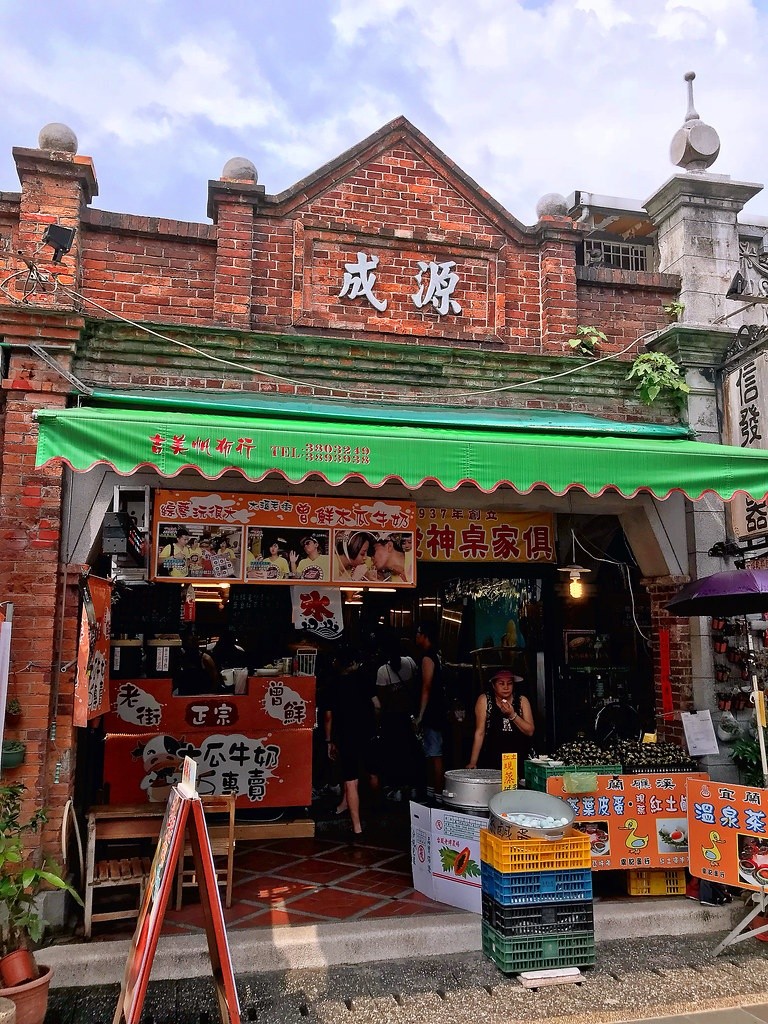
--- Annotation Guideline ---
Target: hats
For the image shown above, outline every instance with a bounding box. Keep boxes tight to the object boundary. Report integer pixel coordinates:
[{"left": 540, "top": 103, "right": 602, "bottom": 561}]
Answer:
[
  {"left": 300, "top": 536, "right": 317, "bottom": 546},
  {"left": 488, "top": 669, "right": 524, "bottom": 682}
]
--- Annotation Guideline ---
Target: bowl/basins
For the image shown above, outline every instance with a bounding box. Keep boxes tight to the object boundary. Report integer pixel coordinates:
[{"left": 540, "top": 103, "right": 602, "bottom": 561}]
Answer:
[
  {"left": 739, "top": 845, "right": 768, "bottom": 885},
  {"left": 548, "top": 761, "right": 564, "bottom": 767},
  {"left": 542, "top": 759, "right": 553, "bottom": 762},
  {"left": 154, "top": 634, "right": 179, "bottom": 639},
  {"left": 254, "top": 669, "right": 278, "bottom": 674},
  {"left": 579, "top": 829, "right": 608, "bottom": 852}
]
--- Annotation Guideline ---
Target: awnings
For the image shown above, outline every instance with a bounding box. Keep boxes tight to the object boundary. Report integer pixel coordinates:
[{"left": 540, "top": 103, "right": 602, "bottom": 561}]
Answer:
[{"left": 31, "top": 386, "right": 768, "bottom": 505}]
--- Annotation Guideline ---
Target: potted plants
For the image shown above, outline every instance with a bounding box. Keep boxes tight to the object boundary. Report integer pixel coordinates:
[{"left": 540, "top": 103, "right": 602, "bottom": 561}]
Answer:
[
  {"left": 749, "top": 708, "right": 765, "bottom": 737},
  {"left": 718, "top": 711, "right": 741, "bottom": 741},
  {"left": 0, "top": 781, "right": 84, "bottom": 1024},
  {"left": 1, "top": 740, "right": 25, "bottom": 769},
  {"left": 5, "top": 699, "right": 21, "bottom": 724}
]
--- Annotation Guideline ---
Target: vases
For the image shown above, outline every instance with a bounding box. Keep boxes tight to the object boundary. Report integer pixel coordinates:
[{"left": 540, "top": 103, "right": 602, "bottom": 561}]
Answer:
[{"left": 0, "top": 949, "right": 39, "bottom": 987}]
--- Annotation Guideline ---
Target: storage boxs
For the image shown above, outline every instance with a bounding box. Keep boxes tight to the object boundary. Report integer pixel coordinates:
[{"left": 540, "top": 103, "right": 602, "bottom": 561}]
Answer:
[{"left": 481, "top": 762, "right": 699, "bottom": 972}]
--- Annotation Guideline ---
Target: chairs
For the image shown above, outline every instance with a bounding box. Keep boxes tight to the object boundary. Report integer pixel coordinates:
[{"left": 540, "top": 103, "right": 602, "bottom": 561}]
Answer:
[{"left": 82, "top": 795, "right": 235, "bottom": 937}]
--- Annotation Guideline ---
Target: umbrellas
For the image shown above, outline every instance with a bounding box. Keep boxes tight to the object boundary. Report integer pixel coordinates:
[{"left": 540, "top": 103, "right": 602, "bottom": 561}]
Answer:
[{"left": 664, "top": 568, "right": 768, "bottom": 651}]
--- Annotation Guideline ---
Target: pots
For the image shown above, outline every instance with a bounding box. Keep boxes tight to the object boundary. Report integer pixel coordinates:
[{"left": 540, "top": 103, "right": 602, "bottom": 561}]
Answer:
[
  {"left": 442, "top": 769, "right": 502, "bottom": 808},
  {"left": 487, "top": 789, "right": 576, "bottom": 842}
]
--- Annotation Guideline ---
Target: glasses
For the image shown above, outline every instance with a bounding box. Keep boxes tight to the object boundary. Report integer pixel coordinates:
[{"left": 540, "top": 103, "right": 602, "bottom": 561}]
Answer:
[{"left": 303, "top": 540, "right": 312, "bottom": 547}]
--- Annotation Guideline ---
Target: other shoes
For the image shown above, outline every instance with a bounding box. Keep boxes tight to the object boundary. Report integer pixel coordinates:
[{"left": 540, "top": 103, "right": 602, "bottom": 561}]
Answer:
[
  {"left": 426, "top": 785, "right": 443, "bottom": 797},
  {"left": 343, "top": 827, "right": 365, "bottom": 841},
  {"left": 329, "top": 806, "right": 349, "bottom": 817}
]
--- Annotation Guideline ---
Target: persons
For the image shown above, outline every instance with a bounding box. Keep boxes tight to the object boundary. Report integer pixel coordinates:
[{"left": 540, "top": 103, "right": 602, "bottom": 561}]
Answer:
[
  {"left": 311, "top": 620, "right": 450, "bottom": 840},
  {"left": 465, "top": 669, "right": 534, "bottom": 776},
  {"left": 182, "top": 640, "right": 218, "bottom": 690},
  {"left": 211, "top": 631, "right": 249, "bottom": 693},
  {"left": 158, "top": 529, "right": 240, "bottom": 578},
  {"left": 334, "top": 530, "right": 412, "bottom": 582},
  {"left": 246, "top": 536, "right": 330, "bottom": 580}
]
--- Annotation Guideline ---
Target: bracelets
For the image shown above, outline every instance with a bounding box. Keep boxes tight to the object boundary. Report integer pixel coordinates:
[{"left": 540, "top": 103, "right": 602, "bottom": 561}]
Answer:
[
  {"left": 510, "top": 712, "right": 517, "bottom": 720},
  {"left": 326, "top": 740, "right": 332, "bottom": 744}
]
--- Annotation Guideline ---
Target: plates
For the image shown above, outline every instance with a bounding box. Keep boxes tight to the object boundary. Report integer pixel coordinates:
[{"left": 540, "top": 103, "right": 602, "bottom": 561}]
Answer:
[
  {"left": 577, "top": 829, "right": 609, "bottom": 855},
  {"left": 529, "top": 761, "right": 547, "bottom": 764},
  {"left": 739, "top": 847, "right": 768, "bottom": 887}
]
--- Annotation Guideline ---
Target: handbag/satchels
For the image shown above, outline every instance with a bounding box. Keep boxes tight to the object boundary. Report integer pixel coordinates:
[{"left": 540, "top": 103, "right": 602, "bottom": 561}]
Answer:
[
  {"left": 157, "top": 543, "right": 175, "bottom": 574},
  {"left": 447, "top": 697, "right": 472, "bottom": 728}
]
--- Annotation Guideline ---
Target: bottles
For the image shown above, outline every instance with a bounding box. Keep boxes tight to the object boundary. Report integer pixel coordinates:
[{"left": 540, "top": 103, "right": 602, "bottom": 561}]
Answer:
[{"left": 410, "top": 715, "right": 424, "bottom": 739}]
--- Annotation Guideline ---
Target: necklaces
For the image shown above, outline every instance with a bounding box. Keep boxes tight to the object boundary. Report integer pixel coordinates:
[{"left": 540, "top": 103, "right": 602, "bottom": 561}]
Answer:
[{"left": 496, "top": 694, "right": 502, "bottom": 703}]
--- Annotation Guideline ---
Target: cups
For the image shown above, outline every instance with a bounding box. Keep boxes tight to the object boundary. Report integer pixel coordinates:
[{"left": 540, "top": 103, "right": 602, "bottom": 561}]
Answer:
[
  {"left": 282, "top": 658, "right": 292, "bottom": 674},
  {"left": 120, "top": 633, "right": 128, "bottom": 640},
  {"left": 233, "top": 668, "right": 245, "bottom": 695}
]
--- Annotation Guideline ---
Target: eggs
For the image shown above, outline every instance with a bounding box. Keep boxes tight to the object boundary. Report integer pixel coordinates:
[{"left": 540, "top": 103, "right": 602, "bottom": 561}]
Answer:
[{"left": 500, "top": 814, "right": 568, "bottom": 829}]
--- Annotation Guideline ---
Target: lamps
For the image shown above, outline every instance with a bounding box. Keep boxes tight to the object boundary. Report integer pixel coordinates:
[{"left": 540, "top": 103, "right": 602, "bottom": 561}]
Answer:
[
  {"left": 726, "top": 270, "right": 748, "bottom": 296},
  {"left": 42, "top": 224, "right": 76, "bottom": 261}
]
